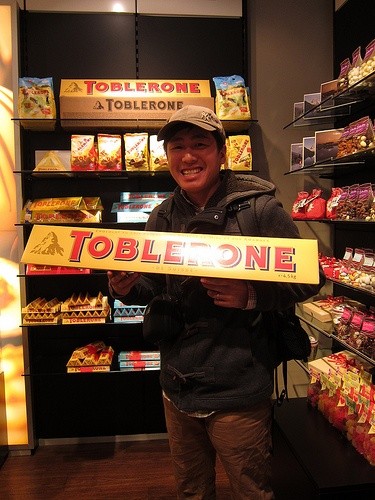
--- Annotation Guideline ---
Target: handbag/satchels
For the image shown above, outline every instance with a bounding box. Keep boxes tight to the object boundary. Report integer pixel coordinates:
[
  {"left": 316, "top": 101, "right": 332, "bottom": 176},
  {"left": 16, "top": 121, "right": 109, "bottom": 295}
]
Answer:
[{"left": 267, "top": 308, "right": 311, "bottom": 366}]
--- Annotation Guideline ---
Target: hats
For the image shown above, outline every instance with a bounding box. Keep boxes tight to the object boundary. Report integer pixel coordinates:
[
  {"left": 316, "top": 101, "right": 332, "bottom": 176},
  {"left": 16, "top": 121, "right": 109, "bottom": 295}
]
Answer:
[{"left": 156, "top": 104, "right": 225, "bottom": 143}]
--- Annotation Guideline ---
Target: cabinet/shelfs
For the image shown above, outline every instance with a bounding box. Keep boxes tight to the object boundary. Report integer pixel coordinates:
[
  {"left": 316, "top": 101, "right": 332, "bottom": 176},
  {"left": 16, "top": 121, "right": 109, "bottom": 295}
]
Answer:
[
  {"left": 10, "top": 118, "right": 260, "bottom": 447},
  {"left": 271, "top": 71, "right": 375, "bottom": 500}
]
